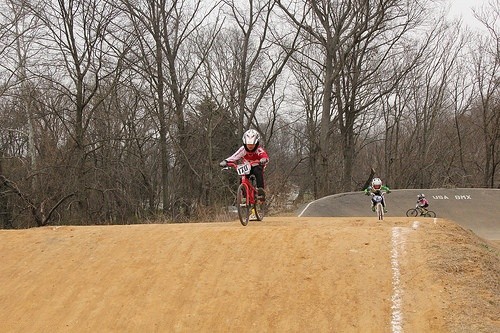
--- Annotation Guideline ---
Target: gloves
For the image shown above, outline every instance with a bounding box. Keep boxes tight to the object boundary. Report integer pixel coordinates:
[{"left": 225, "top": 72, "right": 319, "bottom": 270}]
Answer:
[
  {"left": 219, "top": 160, "right": 228, "bottom": 168},
  {"left": 259, "top": 158, "right": 267, "bottom": 166},
  {"left": 386, "top": 190, "right": 390, "bottom": 194},
  {"left": 364, "top": 192, "right": 370, "bottom": 196}
]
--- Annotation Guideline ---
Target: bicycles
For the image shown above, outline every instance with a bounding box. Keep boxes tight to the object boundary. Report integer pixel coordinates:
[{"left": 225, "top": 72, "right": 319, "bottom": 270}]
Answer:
[
  {"left": 221, "top": 160, "right": 265, "bottom": 226},
  {"left": 365, "top": 191, "right": 391, "bottom": 220},
  {"left": 406, "top": 201, "right": 436, "bottom": 218}
]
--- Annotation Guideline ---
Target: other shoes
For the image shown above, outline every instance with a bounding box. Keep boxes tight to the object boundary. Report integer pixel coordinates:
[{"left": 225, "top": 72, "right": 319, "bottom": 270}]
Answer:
[
  {"left": 422, "top": 211, "right": 428, "bottom": 214},
  {"left": 258, "top": 187, "right": 266, "bottom": 203},
  {"left": 372, "top": 206, "right": 376, "bottom": 212},
  {"left": 384, "top": 210, "right": 388, "bottom": 214}
]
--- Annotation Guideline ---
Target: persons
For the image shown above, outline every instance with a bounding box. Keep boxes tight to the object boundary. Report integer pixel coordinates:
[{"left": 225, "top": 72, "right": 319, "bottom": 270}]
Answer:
[
  {"left": 220, "top": 130, "right": 269, "bottom": 204},
  {"left": 416, "top": 194, "right": 429, "bottom": 214},
  {"left": 364, "top": 178, "right": 390, "bottom": 213}
]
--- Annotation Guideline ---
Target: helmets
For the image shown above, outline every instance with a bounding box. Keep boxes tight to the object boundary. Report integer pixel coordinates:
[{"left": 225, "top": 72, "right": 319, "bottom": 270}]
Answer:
[
  {"left": 241, "top": 129, "right": 260, "bottom": 152},
  {"left": 417, "top": 193, "right": 425, "bottom": 198},
  {"left": 371, "top": 178, "right": 382, "bottom": 190}
]
